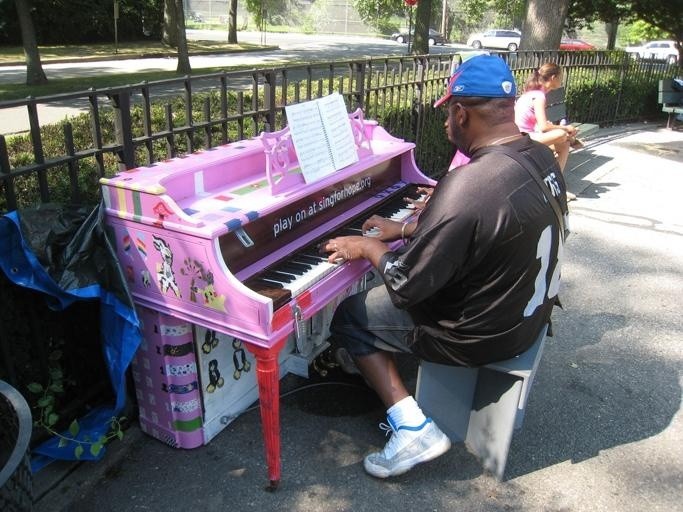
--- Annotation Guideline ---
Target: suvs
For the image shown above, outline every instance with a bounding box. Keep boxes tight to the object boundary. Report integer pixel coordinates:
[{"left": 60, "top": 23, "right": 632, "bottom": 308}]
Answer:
[
  {"left": 467, "top": 27, "right": 521, "bottom": 52},
  {"left": 623, "top": 39, "right": 682, "bottom": 64}
]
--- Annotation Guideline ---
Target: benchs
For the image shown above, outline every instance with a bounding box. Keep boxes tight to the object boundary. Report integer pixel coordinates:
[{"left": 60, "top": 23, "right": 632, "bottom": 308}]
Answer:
[
  {"left": 658, "top": 79, "right": 683, "bottom": 130},
  {"left": 543, "top": 86, "right": 600, "bottom": 151}
]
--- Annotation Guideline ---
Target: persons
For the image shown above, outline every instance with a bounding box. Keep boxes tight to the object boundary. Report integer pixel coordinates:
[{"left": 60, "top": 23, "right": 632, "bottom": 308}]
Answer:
[
  {"left": 315, "top": 54, "right": 575, "bottom": 478},
  {"left": 513, "top": 62, "right": 584, "bottom": 200},
  {"left": 403, "top": 116, "right": 470, "bottom": 210}
]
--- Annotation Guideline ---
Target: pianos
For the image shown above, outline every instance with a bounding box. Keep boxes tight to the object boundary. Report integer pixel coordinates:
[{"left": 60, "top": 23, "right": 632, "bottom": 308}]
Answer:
[{"left": 98, "top": 106, "right": 438, "bottom": 494}]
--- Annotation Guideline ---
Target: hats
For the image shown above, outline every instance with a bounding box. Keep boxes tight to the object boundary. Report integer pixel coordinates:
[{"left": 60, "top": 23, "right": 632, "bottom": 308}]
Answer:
[{"left": 432, "top": 54, "right": 515, "bottom": 108}]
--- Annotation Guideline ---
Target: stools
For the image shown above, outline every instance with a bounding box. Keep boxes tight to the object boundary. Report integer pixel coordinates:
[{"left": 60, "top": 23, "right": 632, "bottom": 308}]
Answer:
[{"left": 400, "top": 319, "right": 551, "bottom": 483}]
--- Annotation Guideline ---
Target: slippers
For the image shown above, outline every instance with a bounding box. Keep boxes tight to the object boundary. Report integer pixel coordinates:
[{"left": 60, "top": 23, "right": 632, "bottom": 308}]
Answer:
[{"left": 568, "top": 138, "right": 584, "bottom": 150}]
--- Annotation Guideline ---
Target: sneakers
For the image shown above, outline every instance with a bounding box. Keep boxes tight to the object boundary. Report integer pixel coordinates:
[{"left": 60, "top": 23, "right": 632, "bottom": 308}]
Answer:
[
  {"left": 362, "top": 416, "right": 450, "bottom": 478},
  {"left": 337, "top": 347, "right": 400, "bottom": 390}
]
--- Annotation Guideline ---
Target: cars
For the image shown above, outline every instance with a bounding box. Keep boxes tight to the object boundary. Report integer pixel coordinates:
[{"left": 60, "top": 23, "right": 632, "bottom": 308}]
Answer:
[
  {"left": 559, "top": 38, "right": 597, "bottom": 51},
  {"left": 390, "top": 27, "right": 445, "bottom": 46}
]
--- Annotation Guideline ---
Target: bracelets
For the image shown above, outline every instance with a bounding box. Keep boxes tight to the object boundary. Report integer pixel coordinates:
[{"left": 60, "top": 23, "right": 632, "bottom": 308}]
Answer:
[{"left": 401, "top": 222, "right": 410, "bottom": 246}]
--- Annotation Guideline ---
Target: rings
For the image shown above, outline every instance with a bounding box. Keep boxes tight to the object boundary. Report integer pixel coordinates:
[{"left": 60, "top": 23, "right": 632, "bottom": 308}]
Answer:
[{"left": 334, "top": 243, "right": 337, "bottom": 251}]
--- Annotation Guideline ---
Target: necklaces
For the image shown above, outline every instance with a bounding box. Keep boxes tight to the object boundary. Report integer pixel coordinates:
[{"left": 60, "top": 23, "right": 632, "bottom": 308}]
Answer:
[{"left": 491, "top": 133, "right": 523, "bottom": 144}]
